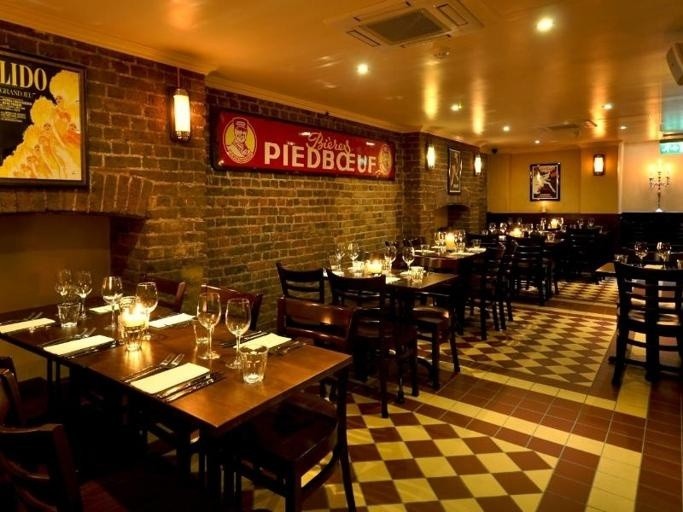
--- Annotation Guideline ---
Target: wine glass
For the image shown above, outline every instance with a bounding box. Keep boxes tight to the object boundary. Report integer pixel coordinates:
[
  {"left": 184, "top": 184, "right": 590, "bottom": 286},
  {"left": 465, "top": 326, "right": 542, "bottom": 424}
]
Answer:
[
  {"left": 49, "top": 268, "right": 91, "bottom": 327},
  {"left": 101, "top": 275, "right": 121, "bottom": 330},
  {"left": 223, "top": 298, "right": 251, "bottom": 369},
  {"left": 197, "top": 291, "right": 220, "bottom": 360},
  {"left": 335, "top": 241, "right": 414, "bottom": 281},
  {"left": 135, "top": 281, "right": 159, "bottom": 340},
  {"left": 434, "top": 230, "right": 465, "bottom": 253}
]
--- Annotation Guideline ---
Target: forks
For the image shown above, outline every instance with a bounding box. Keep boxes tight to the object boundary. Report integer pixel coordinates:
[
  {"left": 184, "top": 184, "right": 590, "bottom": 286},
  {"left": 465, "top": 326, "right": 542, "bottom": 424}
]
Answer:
[
  {"left": 264, "top": 337, "right": 305, "bottom": 355},
  {"left": 120, "top": 352, "right": 183, "bottom": 385},
  {"left": 38, "top": 326, "right": 96, "bottom": 348},
  {"left": 0, "top": 310, "right": 43, "bottom": 327}
]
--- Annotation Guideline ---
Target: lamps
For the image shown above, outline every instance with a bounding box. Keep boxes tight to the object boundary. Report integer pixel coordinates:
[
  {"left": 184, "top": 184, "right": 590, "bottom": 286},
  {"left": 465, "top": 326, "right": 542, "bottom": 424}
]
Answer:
[
  {"left": 648, "top": 159, "right": 671, "bottom": 213},
  {"left": 427, "top": 144, "right": 435, "bottom": 170},
  {"left": 474, "top": 154, "right": 481, "bottom": 175},
  {"left": 593, "top": 154, "right": 606, "bottom": 176},
  {"left": 170, "top": 86, "right": 191, "bottom": 141}
]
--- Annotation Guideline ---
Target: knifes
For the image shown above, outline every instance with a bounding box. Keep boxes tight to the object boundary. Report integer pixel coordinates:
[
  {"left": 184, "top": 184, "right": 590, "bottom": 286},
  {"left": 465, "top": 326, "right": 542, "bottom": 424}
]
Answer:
[
  {"left": 220, "top": 329, "right": 266, "bottom": 349},
  {"left": 65, "top": 339, "right": 122, "bottom": 360},
  {"left": 156, "top": 370, "right": 227, "bottom": 404}
]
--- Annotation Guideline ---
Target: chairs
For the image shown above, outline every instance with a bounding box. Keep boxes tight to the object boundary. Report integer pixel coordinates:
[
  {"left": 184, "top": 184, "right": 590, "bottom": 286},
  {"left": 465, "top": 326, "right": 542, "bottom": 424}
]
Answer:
[
  {"left": 607, "top": 257, "right": 682, "bottom": 394},
  {"left": 18, "top": 221, "right": 610, "bottom": 509}
]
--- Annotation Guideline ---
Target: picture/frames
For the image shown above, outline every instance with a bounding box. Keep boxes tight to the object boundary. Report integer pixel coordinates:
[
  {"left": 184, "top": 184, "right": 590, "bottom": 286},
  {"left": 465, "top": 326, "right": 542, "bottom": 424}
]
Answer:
[
  {"left": 530, "top": 163, "right": 560, "bottom": 201},
  {"left": 0, "top": 47, "right": 89, "bottom": 186},
  {"left": 448, "top": 147, "right": 461, "bottom": 195}
]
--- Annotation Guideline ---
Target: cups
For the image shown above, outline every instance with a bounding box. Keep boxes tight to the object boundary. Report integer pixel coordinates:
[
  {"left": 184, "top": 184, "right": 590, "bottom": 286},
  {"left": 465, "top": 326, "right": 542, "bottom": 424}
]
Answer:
[
  {"left": 419, "top": 245, "right": 429, "bottom": 256},
  {"left": 410, "top": 267, "right": 424, "bottom": 282},
  {"left": 615, "top": 239, "right": 682, "bottom": 271},
  {"left": 192, "top": 317, "right": 211, "bottom": 345},
  {"left": 239, "top": 342, "right": 266, "bottom": 382},
  {"left": 328, "top": 256, "right": 338, "bottom": 270},
  {"left": 119, "top": 297, "right": 145, "bottom": 351},
  {"left": 473, "top": 239, "right": 481, "bottom": 249},
  {"left": 487, "top": 216, "right": 597, "bottom": 240}
]
